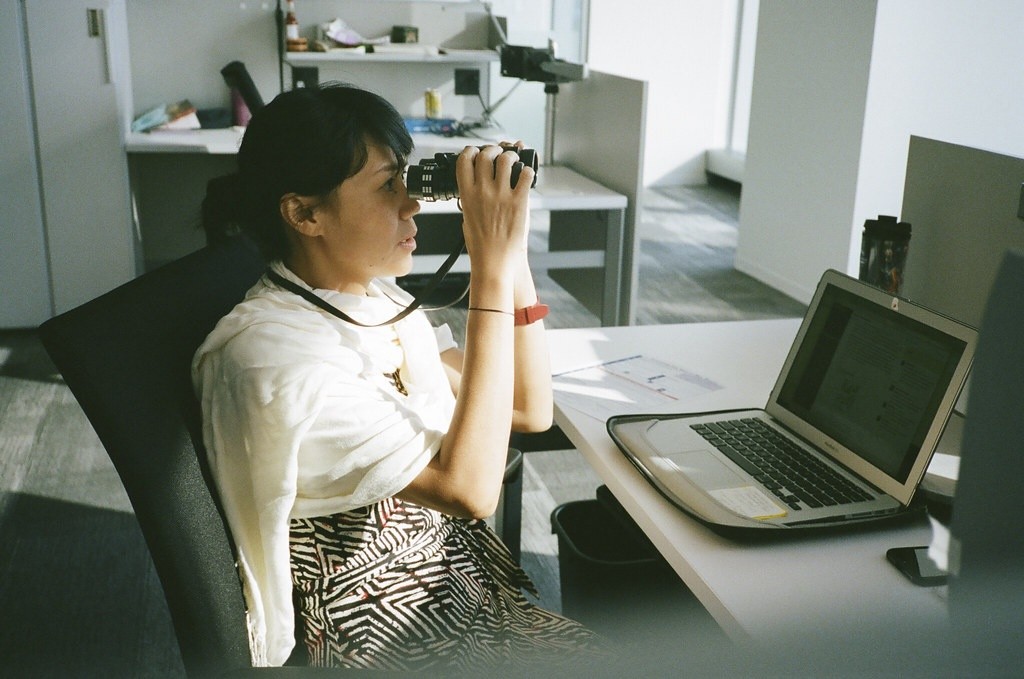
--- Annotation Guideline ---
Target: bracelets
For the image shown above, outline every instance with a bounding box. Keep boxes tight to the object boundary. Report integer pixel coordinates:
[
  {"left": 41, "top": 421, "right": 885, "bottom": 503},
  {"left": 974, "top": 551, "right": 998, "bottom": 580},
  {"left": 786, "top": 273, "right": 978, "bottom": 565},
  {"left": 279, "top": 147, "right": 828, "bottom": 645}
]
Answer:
[
  {"left": 515, "top": 297, "right": 549, "bottom": 327},
  {"left": 467, "top": 307, "right": 515, "bottom": 320}
]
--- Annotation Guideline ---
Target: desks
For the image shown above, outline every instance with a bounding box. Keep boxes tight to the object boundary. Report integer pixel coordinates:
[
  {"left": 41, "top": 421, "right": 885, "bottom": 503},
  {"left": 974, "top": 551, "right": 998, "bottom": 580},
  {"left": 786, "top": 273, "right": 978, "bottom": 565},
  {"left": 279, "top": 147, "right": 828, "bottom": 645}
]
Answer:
[
  {"left": 123, "top": 122, "right": 629, "bottom": 327},
  {"left": 500, "top": 318, "right": 957, "bottom": 652}
]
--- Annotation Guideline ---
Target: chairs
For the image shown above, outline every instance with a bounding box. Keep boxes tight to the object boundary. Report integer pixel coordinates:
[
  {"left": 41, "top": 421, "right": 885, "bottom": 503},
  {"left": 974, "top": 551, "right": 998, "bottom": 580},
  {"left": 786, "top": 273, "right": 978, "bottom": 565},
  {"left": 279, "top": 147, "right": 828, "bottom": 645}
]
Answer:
[{"left": 34, "top": 233, "right": 524, "bottom": 679}]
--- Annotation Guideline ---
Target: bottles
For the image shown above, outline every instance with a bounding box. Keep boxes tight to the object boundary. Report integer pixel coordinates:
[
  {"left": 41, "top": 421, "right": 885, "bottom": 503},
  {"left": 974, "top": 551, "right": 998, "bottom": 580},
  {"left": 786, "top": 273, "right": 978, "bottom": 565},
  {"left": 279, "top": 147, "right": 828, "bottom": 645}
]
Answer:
[
  {"left": 859, "top": 214, "right": 912, "bottom": 294},
  {"left": 285, "top": 0, "right": 298, "bottom": 38}
]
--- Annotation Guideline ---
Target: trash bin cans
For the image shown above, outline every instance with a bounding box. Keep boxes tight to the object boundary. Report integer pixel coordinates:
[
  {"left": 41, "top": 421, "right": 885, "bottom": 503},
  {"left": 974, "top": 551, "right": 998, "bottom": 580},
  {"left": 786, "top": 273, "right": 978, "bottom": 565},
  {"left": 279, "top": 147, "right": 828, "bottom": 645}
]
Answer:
[{"left": 550, "top": 501, "right": 677, "bottom": 642}]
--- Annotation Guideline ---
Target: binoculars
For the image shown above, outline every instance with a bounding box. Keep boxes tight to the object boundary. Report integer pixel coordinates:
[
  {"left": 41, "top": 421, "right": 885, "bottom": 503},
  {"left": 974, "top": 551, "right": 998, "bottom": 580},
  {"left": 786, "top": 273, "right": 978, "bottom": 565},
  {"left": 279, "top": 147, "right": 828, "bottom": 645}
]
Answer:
[{"left": 406, "top": 144, "right": 542, "bottom": 206}]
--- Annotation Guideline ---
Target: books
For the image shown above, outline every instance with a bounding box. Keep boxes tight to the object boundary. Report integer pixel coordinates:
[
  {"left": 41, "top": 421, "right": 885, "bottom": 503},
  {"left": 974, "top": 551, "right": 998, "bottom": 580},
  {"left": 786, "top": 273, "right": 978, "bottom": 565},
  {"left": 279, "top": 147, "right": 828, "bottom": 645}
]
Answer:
[{"left": 130, "top": 98, "right": 201, "bottom": 135}]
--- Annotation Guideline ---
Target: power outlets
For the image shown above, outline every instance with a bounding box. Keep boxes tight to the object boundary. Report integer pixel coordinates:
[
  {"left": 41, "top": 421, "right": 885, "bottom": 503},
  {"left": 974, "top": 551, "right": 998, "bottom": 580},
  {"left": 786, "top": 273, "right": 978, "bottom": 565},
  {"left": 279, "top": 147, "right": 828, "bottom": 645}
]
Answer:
[
  {"left": 456, "top": 68, "right": 480, "bottom": 96},
  {"left": 290, "top": 66, "right": 319, "bottom": 93}
]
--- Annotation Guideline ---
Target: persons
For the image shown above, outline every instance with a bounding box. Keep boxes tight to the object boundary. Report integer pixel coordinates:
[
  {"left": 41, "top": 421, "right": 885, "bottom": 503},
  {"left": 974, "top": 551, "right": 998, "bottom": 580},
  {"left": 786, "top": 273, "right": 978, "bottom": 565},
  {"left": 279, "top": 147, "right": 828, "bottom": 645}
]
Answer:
[{"left": 193, "top": 84, "right": 623, "bottom": 678}]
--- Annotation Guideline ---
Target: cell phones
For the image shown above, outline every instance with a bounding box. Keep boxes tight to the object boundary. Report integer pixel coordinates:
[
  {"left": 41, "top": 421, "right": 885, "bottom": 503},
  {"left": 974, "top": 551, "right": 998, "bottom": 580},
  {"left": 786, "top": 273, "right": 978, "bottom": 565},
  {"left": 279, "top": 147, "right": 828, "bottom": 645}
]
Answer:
[{"left": 886, "top": 546, "right": 949, "bottom": 587}]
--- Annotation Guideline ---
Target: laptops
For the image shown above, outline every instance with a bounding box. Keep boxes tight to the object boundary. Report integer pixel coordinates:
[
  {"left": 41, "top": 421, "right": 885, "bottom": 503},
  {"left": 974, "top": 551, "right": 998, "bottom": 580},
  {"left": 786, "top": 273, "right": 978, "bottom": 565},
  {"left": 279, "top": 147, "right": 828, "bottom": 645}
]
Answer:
[{"left": 610, "top": 269, "right": 983, "bottom": 525}]
